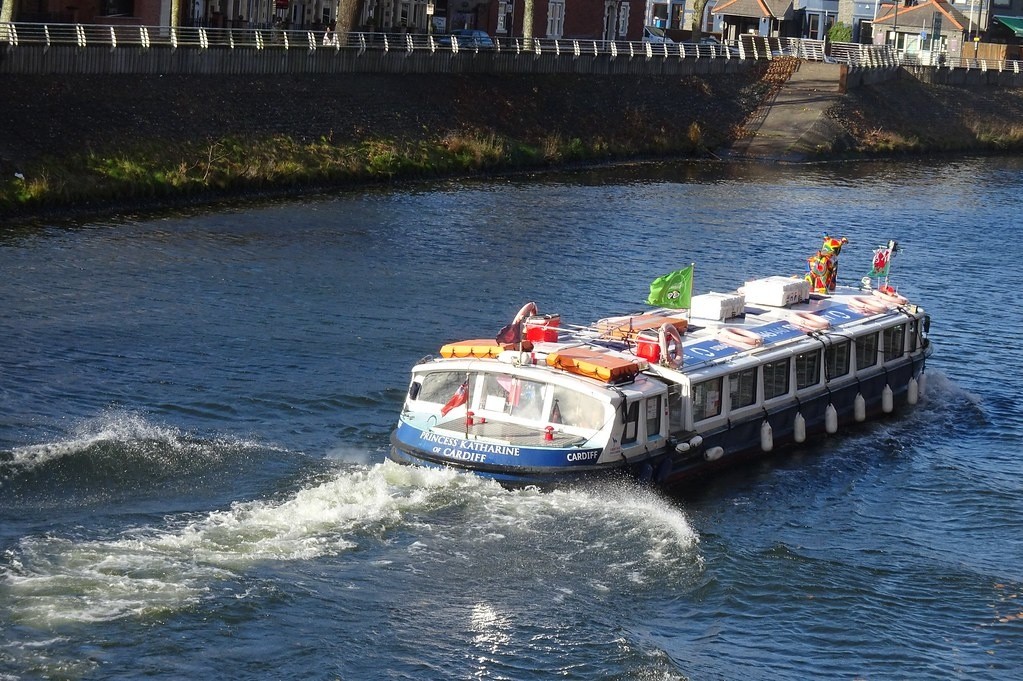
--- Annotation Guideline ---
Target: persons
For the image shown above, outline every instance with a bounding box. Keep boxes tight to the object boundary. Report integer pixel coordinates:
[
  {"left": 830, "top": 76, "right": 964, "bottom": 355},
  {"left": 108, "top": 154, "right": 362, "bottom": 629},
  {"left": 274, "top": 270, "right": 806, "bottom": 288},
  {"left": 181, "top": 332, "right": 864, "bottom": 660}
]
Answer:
[
  {"left": 391, "top": 22, "right": 402, "bottom": 45},
  {"left": 271, "top": 17, "right": 283, "bottom": 43}
]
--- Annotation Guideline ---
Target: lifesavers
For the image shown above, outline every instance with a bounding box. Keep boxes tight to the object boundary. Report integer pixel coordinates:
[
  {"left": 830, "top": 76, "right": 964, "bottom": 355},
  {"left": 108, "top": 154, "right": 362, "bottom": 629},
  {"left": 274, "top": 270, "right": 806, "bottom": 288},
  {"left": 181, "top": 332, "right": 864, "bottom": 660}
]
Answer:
[
  {"left": 660, "top": 322, "right": 684, "bottom": 369},
  {"left": 511, "top": 302, "right": 538, "bottom": 340},
  {"left": 848, "top": 294, "right": 887, "bottom": 313},
  {"left": 787, "top": 310, "right": 830, "bottom": 329},
  {"left": 720, "top": 326, "right": 763, "bottom": 345},
  {"left": 871, "top": 290, "right": 909, "bottom": 305}
]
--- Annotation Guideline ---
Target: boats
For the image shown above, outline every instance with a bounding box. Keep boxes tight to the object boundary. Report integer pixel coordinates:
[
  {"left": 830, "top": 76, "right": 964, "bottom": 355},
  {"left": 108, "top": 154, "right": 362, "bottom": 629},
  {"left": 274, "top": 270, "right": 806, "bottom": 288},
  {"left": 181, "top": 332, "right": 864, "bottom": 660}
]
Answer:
[{"left": 390, "top": 237, "right": 934, "bottom": 500}]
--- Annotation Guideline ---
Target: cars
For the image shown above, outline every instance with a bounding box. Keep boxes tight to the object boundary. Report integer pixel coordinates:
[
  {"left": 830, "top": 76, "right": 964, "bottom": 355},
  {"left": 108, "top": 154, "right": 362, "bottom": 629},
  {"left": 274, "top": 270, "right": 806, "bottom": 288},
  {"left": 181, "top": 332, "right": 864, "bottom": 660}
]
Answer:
[
  {"left": 439, "top": 29, "right": 495, "bottom": 49},
  {"left": 679, "top": 36, "right": 740, "bottom": 55},
  {"left": 645, "top": 26, "right": 676, "bottom": 43}
]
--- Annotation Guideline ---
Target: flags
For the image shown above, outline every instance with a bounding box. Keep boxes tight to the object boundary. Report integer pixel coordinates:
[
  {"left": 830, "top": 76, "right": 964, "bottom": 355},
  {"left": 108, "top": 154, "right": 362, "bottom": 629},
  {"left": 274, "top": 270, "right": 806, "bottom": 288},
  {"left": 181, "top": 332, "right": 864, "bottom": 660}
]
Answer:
[
  {"left": 867, "top": 249, "right": 890, "bottom": 278},
  {"left": 440, "top": 379, "right": 469, "bottom": 417},
  {"left": 645, "top": 266, "right": 692, "bottom": 309}
]
